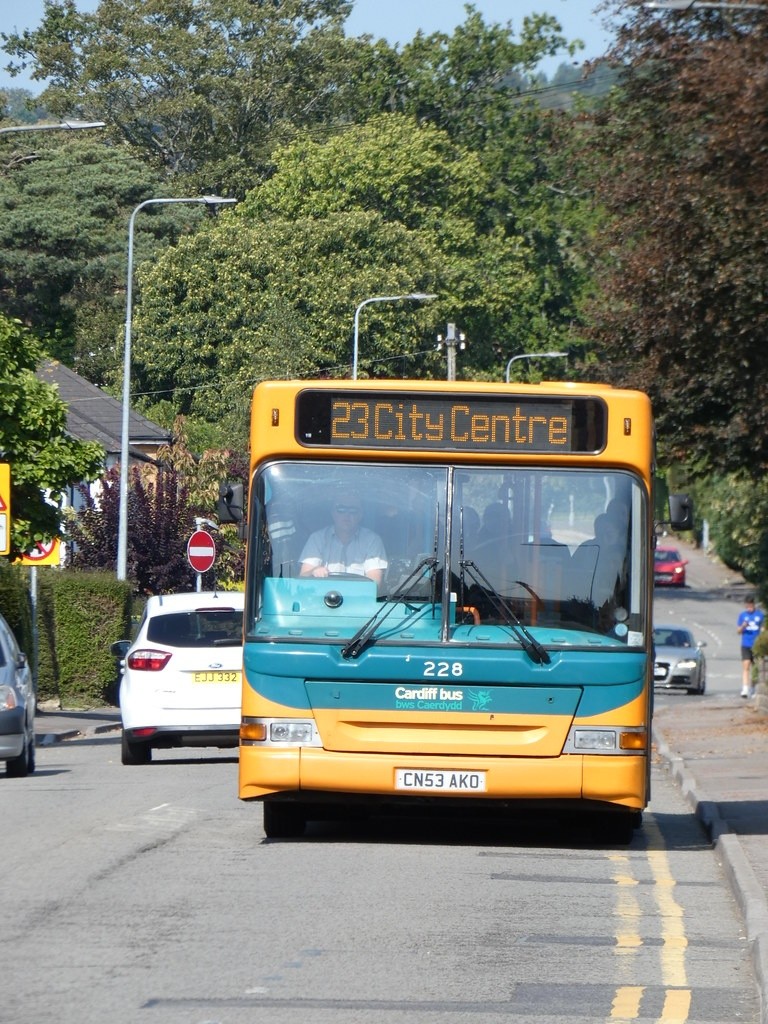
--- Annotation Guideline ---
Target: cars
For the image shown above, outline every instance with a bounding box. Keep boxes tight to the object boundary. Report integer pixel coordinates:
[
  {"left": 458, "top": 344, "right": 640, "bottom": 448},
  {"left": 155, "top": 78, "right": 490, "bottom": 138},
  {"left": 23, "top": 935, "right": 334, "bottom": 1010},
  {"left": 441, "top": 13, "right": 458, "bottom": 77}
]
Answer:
[
  {"left": 0, "top": 614, "right": 38, "bottom": 778},
  {"left": 651, "top": 547, "right": 689, "bottom": 588},
  {"left": 647, "top": 625, "right": 708, "bottom": 695},
  {"left": 111, "top": 591, "right": 247, "bottom": 765}
]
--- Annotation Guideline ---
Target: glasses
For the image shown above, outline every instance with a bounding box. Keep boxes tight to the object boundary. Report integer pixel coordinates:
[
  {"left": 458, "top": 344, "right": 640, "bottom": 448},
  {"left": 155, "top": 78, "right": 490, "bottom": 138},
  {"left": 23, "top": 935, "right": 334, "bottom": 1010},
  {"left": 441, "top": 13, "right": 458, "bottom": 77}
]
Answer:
[{"left": 336, "top": 506, "right": 358, "bottom": 513}]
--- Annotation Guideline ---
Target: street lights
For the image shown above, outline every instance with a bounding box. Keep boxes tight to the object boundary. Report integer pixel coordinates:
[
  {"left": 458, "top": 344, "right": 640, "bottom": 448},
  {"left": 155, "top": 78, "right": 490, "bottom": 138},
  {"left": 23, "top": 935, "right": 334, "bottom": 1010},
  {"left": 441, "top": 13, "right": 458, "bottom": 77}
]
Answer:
[
  {"left": 352, "top": 293, "right": 439, "bottom": 381},
  {"left": 115, "top": 196, "right": 238, "bottom": 583},
  {"left": 195, "top": 516, "right": 220, "bottom": 594},
  {"left": 507, "top": 350, "right": 569, "bottom": 383}
]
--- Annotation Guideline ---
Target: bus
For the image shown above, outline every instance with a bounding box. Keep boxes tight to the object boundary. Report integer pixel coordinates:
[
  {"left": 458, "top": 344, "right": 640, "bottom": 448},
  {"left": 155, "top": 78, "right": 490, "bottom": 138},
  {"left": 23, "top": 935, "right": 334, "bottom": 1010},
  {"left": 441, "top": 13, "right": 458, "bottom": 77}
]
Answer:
[{"left": 216, "top": 382, "right": 697, "bottom": 841}]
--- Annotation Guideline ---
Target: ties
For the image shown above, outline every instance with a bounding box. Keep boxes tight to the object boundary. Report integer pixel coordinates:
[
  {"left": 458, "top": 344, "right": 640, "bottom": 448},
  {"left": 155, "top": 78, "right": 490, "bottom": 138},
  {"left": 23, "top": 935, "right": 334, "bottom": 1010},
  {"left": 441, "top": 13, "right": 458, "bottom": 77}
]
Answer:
[{"left": 340, "top": 546, "right": 347, "bottom": 576}]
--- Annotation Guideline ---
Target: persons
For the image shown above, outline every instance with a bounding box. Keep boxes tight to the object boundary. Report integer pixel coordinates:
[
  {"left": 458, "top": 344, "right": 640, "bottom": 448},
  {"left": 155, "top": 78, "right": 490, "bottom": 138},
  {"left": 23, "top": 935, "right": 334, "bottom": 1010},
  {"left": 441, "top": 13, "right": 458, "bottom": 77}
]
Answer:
[
  {"left": 298, "top": 495, "right": 388, "bottom": 588},
  {"left": 736, "top": 595, "right": 767, "bottom": 699},
  {"left": 463, "top": 498, "right": 628, "bottom": 602}
]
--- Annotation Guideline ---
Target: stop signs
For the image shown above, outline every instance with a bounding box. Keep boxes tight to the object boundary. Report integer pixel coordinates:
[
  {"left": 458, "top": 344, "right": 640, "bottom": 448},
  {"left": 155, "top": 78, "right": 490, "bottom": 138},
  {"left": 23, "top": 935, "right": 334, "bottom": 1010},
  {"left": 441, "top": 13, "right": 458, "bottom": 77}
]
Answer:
[{"left": 188, "top": 530, "right": 216, "bottom": 572}]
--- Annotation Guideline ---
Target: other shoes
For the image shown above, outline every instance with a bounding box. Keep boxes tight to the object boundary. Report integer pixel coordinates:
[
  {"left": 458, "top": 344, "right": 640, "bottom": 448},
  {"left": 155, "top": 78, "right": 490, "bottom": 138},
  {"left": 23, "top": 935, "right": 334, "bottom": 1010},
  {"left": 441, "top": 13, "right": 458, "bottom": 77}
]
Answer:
[
  {"left": 740, "top": 686, "right": 748, "bottom": 695},
  {"left": 751, "top": 693, "right": 756, "bottom": 699}
]
